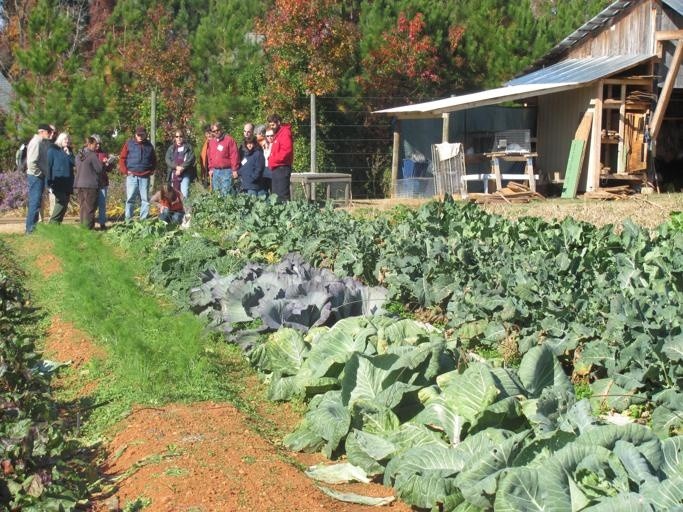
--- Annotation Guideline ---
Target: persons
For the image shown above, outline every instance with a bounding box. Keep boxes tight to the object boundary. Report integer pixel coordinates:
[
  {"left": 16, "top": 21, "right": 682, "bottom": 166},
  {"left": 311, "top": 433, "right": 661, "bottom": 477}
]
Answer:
[{"left": 16, "top": 114, "right": 294, "bottom": 234}]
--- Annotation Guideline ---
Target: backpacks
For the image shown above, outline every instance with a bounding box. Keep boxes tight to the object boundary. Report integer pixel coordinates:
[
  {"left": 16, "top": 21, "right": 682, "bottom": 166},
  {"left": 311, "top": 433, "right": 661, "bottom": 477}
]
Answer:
[{"left": 15, "top": 144, "right": 27, "bottom": 177}]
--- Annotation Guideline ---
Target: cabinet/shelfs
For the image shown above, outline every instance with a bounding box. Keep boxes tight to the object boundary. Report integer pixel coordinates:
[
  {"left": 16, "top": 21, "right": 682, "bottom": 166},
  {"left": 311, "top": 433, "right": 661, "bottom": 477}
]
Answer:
[{"left": 595, "top": 77, "right": 654, "bottom": 181}]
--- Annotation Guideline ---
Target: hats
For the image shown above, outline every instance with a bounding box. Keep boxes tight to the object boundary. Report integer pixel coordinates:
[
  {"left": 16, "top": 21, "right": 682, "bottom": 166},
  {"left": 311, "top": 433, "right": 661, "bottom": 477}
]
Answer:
[
  {"left": 38, "top": 124, "right": 53, "bottom": 131},
  {"left": 136, "top": 126, "right": 145, "bottom": 135},
  {"left": 265, "top": 129, "right": 273, "bottom": 135}
]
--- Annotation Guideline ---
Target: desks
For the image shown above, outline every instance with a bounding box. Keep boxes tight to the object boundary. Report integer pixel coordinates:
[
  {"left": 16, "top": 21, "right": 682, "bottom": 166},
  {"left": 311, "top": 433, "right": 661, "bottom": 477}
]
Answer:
[
  {"left": 284, "top": 170, "right": 352, "bottom": 211},
  {"left": 459, "top": 149, "right": 540, "bottom": 197}
]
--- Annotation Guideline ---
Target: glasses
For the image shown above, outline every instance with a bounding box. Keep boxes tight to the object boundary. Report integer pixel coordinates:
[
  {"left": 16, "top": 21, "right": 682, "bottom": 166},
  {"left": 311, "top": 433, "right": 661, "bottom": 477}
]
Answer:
[{"left": 175, "top": 135, "right": 182, "bottom": 138}]
